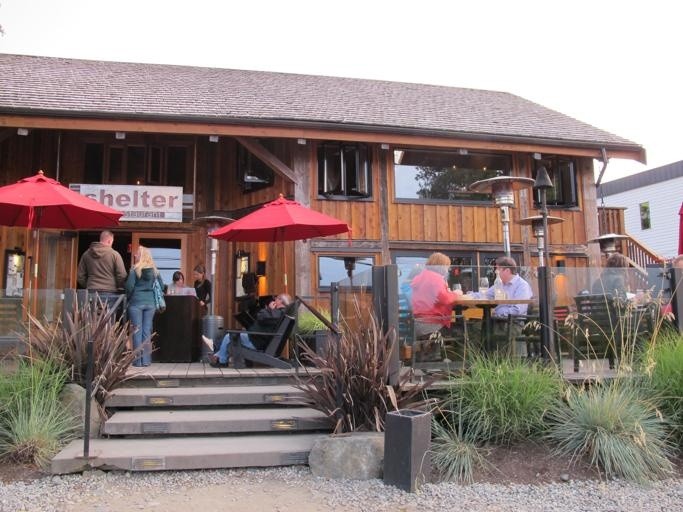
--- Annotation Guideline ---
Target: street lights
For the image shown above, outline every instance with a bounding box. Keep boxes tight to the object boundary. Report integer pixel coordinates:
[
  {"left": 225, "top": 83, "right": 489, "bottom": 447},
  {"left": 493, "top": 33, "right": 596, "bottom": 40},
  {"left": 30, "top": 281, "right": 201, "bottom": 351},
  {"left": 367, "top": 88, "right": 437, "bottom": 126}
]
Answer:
[{"left": 530, "top": 158, "right": 564, "bottom": 377}]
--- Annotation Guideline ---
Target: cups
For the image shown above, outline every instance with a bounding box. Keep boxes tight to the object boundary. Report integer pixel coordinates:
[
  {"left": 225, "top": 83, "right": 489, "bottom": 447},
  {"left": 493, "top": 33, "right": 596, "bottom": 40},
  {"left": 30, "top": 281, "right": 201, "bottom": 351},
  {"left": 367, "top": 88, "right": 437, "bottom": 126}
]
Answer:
[{"left": 625, "top": 287, "right": 654, "bottom": 302}]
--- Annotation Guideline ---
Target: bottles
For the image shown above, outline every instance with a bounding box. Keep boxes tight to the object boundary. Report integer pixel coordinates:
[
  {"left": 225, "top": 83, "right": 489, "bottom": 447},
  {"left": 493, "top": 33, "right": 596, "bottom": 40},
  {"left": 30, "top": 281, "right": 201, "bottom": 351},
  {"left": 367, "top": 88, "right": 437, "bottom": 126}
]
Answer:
[{"left": 494, "top": 270, "right": 506, "bottom": 299}]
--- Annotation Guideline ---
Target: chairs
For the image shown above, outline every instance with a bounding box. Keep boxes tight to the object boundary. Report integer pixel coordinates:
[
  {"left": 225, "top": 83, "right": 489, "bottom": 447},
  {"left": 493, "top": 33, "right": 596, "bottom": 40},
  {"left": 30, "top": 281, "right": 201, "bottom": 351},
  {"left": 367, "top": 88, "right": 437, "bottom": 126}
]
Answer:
[
  {"left": 0, "top": 299, "right": 29, "bottom": 375},
  {"left": 225, "top": 314, "right": 296, "bottom": 369},
  {"left": 397, "top": 293, "right": 676, "bottom": 383}
]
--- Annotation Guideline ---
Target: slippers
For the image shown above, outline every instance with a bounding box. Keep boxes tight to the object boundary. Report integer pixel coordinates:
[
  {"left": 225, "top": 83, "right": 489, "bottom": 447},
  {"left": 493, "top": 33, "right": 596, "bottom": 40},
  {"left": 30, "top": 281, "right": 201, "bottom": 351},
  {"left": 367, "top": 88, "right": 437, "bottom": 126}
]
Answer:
[{"left": 200, "top": 335, "right": 215, "bottom": 352}]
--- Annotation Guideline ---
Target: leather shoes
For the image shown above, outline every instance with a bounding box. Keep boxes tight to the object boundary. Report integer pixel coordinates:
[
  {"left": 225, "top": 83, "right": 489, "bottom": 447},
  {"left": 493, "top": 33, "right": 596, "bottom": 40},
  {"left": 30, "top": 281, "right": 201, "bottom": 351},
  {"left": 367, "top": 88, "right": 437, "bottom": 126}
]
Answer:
[{"left": 210, "top": 359, "right": 229, "bottom": 368}]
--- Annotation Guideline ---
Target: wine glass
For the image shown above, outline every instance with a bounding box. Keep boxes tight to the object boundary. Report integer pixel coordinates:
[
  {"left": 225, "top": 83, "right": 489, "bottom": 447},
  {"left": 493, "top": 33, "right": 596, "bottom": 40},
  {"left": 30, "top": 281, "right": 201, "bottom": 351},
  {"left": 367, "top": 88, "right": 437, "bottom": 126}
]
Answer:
[{"left": 451, "top": 276, "right": 489, "bottom": 300}]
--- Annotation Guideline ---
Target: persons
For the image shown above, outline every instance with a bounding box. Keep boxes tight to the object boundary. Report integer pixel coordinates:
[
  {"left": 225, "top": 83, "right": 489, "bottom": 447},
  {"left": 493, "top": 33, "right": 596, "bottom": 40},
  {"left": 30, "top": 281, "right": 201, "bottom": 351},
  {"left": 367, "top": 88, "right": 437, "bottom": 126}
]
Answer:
[
  {"left": 168, "top": 271, "right": 190, "bottom": 295},
  {"left": 409, "top": 252, "right": 464, "bottom": 340},
  {"left": 672, "top": 254, "right": 683, "bottom": 272},
  {"left": 192, "top": 263, "right": 211, "bottom": 313},
  {"left": 591, "top": 252, "right": 644, "bottom": 333},
  {"left": 208, "top": 293, "right": 292, "bottom": 368},
  {"left": 77, "top": 231, "right": 128, "bottom": 329},
  {"left": 399, "top": 268, "right": 422, "bottom": 319},
  {"left": 125, "top": 244, "right": 164, "bottom": 367},
  {"left": 468, "top": 256, "right": 533, "bottom": 337}
]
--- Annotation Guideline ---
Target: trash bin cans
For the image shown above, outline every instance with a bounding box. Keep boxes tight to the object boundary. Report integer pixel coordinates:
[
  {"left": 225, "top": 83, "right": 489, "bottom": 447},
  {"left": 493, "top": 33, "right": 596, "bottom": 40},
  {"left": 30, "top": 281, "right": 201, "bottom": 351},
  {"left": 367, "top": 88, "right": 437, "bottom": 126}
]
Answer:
[{"left": 200, "top": 314, "right": 224, "bottom": 363}]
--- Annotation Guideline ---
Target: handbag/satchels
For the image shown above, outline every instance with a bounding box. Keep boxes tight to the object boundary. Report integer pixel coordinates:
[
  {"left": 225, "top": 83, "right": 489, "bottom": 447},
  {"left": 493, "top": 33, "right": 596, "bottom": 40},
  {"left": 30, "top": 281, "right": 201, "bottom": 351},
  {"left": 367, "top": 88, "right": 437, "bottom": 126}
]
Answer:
[{"left": 150, "top": 267, "right": 167, "bottom": 313}]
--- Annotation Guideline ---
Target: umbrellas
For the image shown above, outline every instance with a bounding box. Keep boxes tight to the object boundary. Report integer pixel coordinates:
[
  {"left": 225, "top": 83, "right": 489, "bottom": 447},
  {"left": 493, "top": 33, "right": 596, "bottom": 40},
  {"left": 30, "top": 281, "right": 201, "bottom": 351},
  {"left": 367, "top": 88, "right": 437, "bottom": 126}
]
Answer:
[
  {"left": 0, "top": 169, "right": 124, "bottom": 322},
  {"left": 207, "top": 192, "right": 352, "bottom": 296},
  {"left": 677, "top": 202, "right": 683, "bottom": 256}
]
